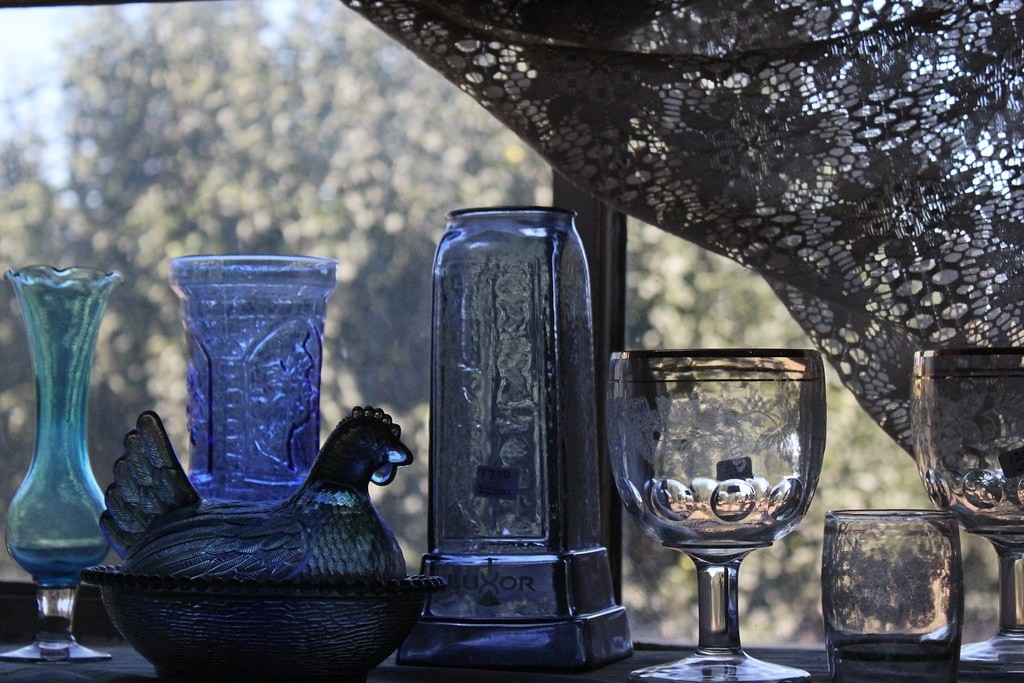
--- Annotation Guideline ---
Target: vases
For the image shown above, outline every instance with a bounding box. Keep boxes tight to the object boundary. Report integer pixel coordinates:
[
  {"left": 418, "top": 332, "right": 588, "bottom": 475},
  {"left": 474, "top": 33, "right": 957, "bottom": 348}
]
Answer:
[{"left": 5, "top": 206, "right": 634, "bottom": 673}]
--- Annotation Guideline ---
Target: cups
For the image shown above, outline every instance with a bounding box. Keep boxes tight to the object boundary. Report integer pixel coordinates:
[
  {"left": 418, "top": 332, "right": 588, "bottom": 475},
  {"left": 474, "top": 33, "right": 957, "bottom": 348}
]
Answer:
[
  {"left": 820, "top": 509, "right": 962, "bottom": 680},
  {"left": 167, "top": 254, "right": 336, "bottom": 509}
]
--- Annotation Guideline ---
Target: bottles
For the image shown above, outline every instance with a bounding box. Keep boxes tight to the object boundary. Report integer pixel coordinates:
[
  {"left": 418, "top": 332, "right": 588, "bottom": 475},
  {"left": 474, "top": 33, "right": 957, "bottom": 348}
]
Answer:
[{"left": 392, "top": 204, "right": 633, "bottom": 673}]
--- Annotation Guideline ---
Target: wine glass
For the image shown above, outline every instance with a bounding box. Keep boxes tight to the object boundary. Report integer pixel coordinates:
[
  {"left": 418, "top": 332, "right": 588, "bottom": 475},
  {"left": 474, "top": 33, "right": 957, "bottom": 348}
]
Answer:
[
  {"left": 910, "top": 346, "right": 1024, "bottom": 666},
  {"left": 604, "top": 349, "right": 827, "bottom": 683},
  {"left": 0, "top": 266, "right": 123, "bottom": 660}
]
